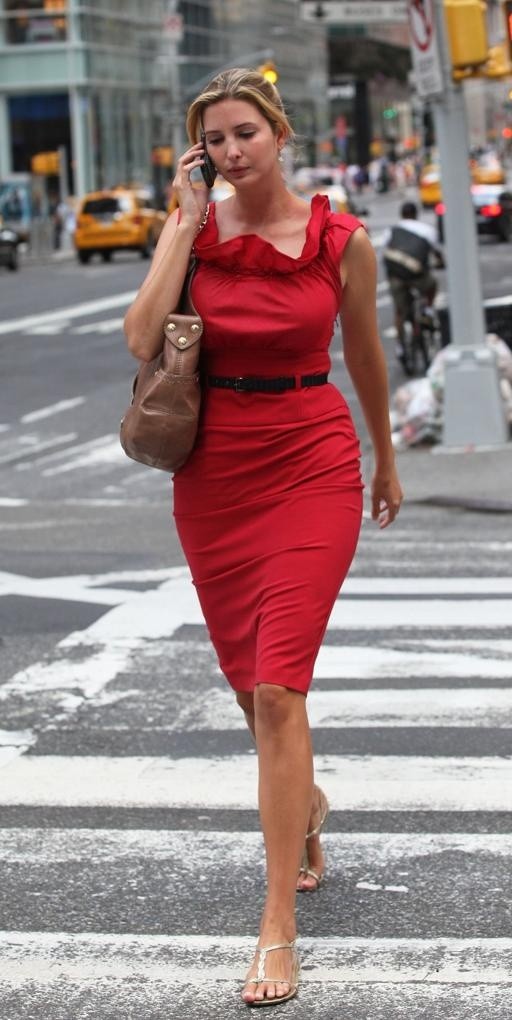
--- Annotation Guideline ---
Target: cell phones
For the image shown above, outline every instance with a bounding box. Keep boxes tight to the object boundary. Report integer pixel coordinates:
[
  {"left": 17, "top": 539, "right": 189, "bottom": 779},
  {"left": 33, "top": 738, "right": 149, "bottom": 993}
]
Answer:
[{"left": 200, "top": 130, "right": 217, "bottom": 188}]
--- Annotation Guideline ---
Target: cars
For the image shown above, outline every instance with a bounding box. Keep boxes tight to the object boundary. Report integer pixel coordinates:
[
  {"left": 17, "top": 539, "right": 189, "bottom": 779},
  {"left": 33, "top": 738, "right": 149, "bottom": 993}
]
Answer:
[
  {"left": 419, "top": 146, "right": 512, "bottom": 244},
  {"left": 71, "top": 189, "right": 171, "bottom": 266}
]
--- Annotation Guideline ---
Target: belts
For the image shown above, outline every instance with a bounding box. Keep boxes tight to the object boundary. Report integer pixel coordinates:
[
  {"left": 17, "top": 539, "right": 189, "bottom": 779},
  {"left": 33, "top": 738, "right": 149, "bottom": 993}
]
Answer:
[{"left": 202, "top": 370, "right": 329, "bottom": 394}]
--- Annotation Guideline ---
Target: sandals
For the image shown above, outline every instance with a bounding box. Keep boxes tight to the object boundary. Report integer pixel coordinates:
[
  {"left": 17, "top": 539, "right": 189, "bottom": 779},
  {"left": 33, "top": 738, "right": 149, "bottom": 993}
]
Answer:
[
  {"left": 242, "top": 927, "right": 300, "bottom": 1008},
  {"left": 290, "top": 789, "right": 329, "bottom": 895}
]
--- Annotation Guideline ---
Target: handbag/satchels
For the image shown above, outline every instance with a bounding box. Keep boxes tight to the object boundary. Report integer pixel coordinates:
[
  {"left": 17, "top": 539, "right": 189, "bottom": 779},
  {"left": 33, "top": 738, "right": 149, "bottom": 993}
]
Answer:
[{"left": 118, "top": 253, "right": 205, "bottom": 473}]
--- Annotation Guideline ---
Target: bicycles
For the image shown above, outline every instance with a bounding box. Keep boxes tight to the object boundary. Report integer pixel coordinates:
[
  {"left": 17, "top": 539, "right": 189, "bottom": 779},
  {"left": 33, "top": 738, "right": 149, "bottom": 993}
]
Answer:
[{"left": 393, "top": 259, "right": 449, "bottom": 377}]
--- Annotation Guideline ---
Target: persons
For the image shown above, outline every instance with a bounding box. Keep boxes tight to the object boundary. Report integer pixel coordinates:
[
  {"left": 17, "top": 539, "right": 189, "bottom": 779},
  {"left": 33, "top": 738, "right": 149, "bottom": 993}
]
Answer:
[
  {"left": 378, "top": 201, "right": 447, "bottom": 362},
  {"left": 120, "top": 67, "right": 406, "bottom": 1009},
  {"left": 331, "top": 154, "right": 427, "bottom": 195}
]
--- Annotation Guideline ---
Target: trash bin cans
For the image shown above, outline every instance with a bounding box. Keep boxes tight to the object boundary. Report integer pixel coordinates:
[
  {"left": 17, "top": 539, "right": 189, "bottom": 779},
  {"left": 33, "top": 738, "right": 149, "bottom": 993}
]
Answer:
[{"left": 433, "top": 295, "right": 511, "bottom": 350}]
